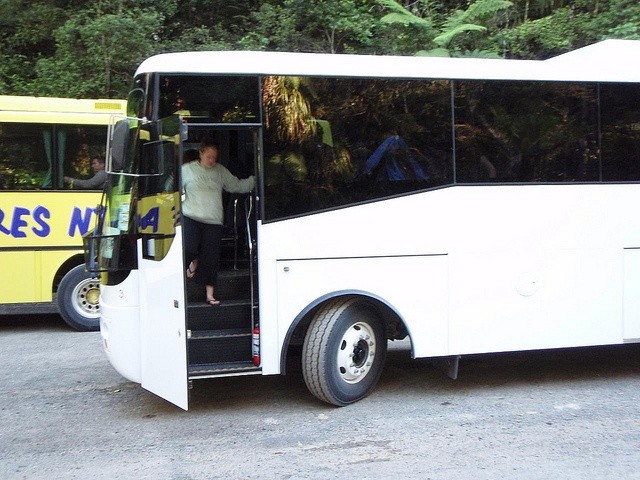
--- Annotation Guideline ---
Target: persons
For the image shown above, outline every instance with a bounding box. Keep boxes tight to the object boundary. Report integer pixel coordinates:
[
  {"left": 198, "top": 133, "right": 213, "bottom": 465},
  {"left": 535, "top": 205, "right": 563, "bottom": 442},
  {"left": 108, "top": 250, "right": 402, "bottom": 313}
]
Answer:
[
  {"left": 163, "top": 138, "right": 255, "bottom": 307},
  {"left": 364, "top": 112, "right": 528, "bottom": 194},
  {"left": 63, "top": 155, "right": 107, "bottom": 189},
  {"left": 165, "top": 149, "right": 199, "bottom": 192},
  {"left": 52, "top": 132, "right": 85, "bottom": 185}
]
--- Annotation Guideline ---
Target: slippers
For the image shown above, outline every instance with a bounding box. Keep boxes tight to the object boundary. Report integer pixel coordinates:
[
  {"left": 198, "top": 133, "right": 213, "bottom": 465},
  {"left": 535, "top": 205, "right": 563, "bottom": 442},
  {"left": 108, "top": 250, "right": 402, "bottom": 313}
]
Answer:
[
  {"left": 207, "top": 296, "right": 221, "bottom": 305},
  {"left": 186, "top": 260, "right": 197, "bottom": 278}
]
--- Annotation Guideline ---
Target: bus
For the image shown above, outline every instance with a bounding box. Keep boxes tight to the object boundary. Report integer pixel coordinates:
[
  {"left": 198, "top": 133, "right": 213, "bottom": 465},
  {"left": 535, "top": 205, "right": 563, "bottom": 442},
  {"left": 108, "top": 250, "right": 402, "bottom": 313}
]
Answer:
[
  {"left": 0, "top": 95, "right": 133, "bottom": 331},
  {"left": 82, "top": 37, "right": 640, "bottom": 414}
]
